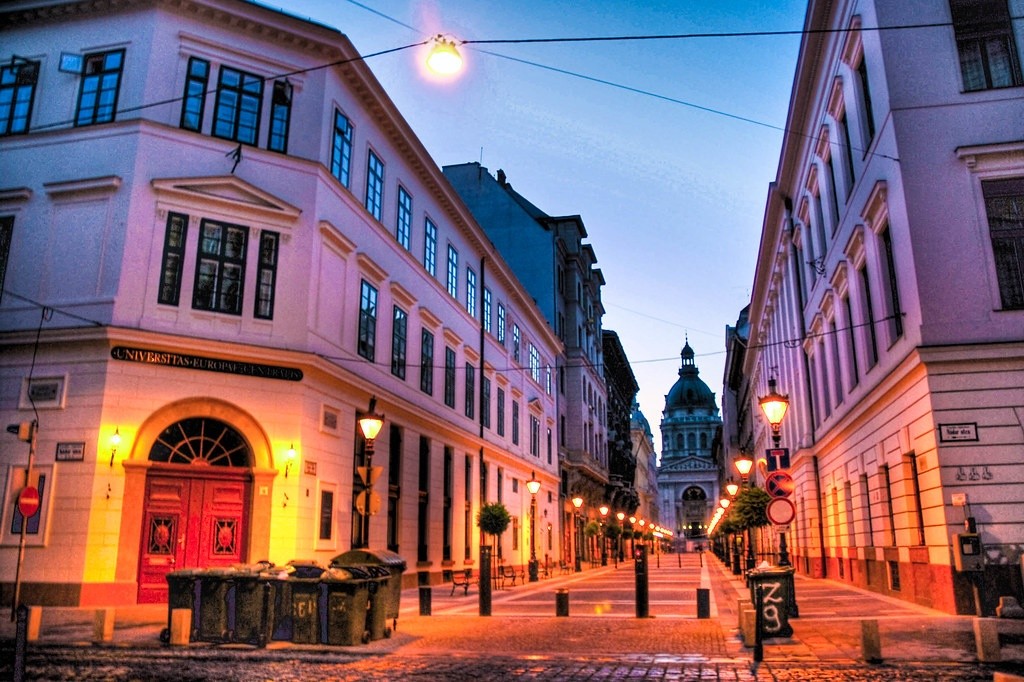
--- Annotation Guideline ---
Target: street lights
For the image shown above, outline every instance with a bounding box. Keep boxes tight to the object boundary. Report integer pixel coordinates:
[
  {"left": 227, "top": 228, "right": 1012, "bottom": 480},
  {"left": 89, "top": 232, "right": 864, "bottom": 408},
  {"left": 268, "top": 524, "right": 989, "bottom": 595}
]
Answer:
[
  {"left": 650, "top": 523, "right": 655, "bottom": 555},
  {"left": 616, "top": 511, "right": 625, "bottom": 562},
  {"left": 639, "top": 519, "right": 648, "bottom": 548},
  {"left": 719, "top": 492, "right": 732, "bottom": 567},
  {"left": 599, "top": 502, "right": 608, "bottom": 566},
  {"left": 355, "top": 396, "right": 386, "bottom": 548},
  {"left": 629, "top": 516, "right": 635, "bottom": 559},
  {"left": 726, "top": 477, "right": 742, "bottom": 575},
  {"left": 757, "top": 374, "right": 803, "bottom": 617},
  {"left": 734, "top": 445, "right": 757, "bottom": 587},
  {"left": 572, "top": 489, "right": 582, "bottom": 572},
  {"left": 525, "top": 471, "right": 542, "bottom": 582}
]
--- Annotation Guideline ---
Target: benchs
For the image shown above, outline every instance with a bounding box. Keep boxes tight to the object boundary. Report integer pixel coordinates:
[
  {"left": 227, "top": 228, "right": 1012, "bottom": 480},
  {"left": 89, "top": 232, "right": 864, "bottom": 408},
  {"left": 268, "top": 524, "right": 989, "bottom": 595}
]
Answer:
[{"left": 450, "top": 559, "right": 601, "bottom": 597}]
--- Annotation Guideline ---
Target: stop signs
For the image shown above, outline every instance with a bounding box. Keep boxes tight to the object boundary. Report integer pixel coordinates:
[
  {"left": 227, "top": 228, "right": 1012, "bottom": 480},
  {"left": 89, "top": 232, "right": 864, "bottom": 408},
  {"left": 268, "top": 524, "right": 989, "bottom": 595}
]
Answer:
[{"left": 18, "top": 486, "right": 41, "bottom": 518}]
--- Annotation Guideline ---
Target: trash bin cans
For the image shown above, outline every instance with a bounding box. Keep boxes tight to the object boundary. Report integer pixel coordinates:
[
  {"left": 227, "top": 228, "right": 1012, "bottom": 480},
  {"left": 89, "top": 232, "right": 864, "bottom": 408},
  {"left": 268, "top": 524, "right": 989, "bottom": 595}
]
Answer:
[
  {"left": 330, "top": 547, "right": 409, "bottom": 631},
  {"left": 160, "top": 560, "right": 392, "bottom": 649},
  {"left": 746, "top": 565, "right": 796, "bottom": 640}
]
